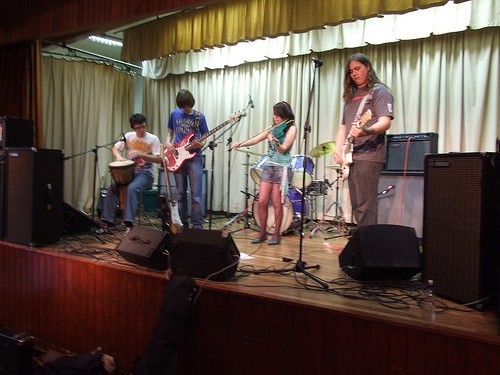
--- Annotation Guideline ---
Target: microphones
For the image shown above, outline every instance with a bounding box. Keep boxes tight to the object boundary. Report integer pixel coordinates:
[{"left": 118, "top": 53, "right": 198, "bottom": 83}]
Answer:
[
  {"left": 122, "top": 133, "right": 126, "bottom": 142},
  {"left": 383, "top": 185, "right": 395, "bottom": 194},
  {"left": 249, "top": 96, "right": 254, "bottom": 108},
  {"left": 312, "top": 57, "right": 323, "bottom": 67}
]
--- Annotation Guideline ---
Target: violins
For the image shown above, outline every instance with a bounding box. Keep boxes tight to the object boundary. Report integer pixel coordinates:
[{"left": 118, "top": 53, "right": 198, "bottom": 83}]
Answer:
[{"left": 266, "top": 121, "right": 288, "bottom": 144}]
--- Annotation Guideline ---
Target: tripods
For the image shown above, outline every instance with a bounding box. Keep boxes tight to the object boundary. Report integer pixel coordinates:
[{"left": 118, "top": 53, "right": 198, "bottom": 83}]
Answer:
[
  {"left": 64, "top": 138, "right": 122, "bottom": 243},
  {"left": 229, "top": 64, "right": 345, "bottom": 289}
]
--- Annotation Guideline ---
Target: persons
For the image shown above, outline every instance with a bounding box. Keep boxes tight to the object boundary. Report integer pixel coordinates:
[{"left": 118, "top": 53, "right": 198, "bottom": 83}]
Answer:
[
  {"left": 100, "top": 114, "right": 161, "bottom": 235},
  {"left": 232, "top": 101, "right": 296, "bottom": 245},
  {"left": 334, "top": 53, "right": 395, "bottom": 226},
  {"left": 166, "top": 89, "right": 208, "bottom": 230}
]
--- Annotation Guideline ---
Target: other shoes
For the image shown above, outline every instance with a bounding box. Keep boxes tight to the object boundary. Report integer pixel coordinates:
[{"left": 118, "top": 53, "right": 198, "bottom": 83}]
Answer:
[
  {"left": 89, "top": 224, "right": 116, "bottom": 234},
  {"left": 268, "top": 237, "right": 283, "bottom": 244},
  {"left": 250, "top": 235, "right": 267, "bottom": 243}
]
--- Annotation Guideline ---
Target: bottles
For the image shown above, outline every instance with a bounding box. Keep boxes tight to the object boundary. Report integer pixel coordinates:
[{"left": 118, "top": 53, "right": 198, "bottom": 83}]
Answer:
[{"left": 420, "top": 279, "right": 436, "bottom": 321}]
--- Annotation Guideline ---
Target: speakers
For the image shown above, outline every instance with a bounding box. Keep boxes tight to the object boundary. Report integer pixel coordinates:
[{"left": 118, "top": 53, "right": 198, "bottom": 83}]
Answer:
[
  {"left": 116, "top": 225, "right": 172, "bottom": 271},
  {"left": 169, "top": 227, "right": 241, "bottom": 282},
  {"left": 339, "top": 132, "right": 500, "bottom": 313},
  {"left": 0, "top": 116, "right": 64, "bottom": 247}
]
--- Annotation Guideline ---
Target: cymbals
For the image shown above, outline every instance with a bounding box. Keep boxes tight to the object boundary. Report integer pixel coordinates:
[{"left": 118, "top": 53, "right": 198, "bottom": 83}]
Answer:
[
  {"left": 234, "top": 148, "right": 263, "bottom": 156},
  {"left": 241, "top": 162, "right": 259, "bottom": 166},
  {"left": 309, "top": 141, "right": 336, "bottom": 158},
  {"left": 324, "top": 165, "right": 346, "bottom": 169}
]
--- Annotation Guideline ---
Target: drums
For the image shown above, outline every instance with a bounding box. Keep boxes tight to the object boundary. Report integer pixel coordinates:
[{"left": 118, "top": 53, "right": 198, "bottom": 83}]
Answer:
[
  {"left": 252, "top": 187, "right": 310, "bottom": 235},
  {"left": 108, "top": 160, "right": 137, "bottom": 210},
  {"left": 250, "top": 156, "right": 271, "bottom": 186},
  {"left": 304, "top": 180, "right": 328, "bottom": 196},
  {"left": 289, "top": 154, "right": 315, "bottom": 189}
]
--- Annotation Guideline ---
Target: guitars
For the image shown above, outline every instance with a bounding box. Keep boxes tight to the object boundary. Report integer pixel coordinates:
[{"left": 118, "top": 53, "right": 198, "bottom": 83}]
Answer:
[
  {"left": 163, "top": 113, "right": 238, "bottom": 174},
  {"left": 339, "top": 108, "right": 372, "bottom": 181},
  {"left": 162, "top": 156, "right": 184, "bottom": 235}
]
